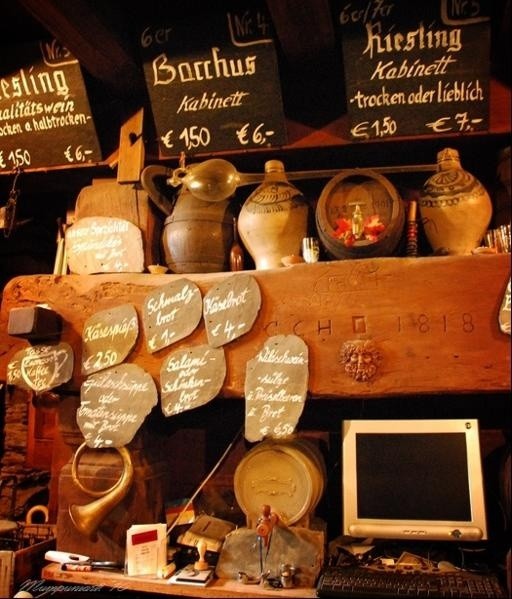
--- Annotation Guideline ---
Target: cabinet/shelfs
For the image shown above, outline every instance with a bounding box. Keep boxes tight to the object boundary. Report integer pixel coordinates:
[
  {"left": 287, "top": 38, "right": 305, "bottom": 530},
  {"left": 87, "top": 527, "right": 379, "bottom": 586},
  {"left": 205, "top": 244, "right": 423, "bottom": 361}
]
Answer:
[{"left": 0, "top": 255, "right": 512, "bottom": 394}]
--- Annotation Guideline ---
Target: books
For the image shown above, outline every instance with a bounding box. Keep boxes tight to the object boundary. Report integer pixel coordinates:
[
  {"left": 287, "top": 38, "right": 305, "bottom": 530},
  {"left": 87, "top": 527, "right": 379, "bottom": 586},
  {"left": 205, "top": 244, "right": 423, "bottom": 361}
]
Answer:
[{"left": 167, "top": 564, "right": 215, "bottom": 588}]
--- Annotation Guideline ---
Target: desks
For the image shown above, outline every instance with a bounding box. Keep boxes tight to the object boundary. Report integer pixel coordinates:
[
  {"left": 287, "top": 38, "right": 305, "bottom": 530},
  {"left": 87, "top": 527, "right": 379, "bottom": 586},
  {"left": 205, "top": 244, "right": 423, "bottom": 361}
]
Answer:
[{"left": 41, "top": 555, "right": 317, "bottom": 599}]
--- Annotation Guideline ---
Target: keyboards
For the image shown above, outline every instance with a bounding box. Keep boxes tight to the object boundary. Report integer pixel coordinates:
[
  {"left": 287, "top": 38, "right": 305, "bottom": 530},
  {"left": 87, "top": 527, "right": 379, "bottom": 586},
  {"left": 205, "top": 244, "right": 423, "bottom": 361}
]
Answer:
[{"left": 316, "top": 566, "right": 504, "bottom": 599}]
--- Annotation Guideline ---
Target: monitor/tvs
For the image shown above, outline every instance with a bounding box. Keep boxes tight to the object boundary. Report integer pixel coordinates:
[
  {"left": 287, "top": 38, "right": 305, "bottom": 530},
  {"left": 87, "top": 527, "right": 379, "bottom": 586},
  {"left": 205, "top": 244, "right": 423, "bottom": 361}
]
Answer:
[{"left": 343, "top": 420, "right": 488, "bottom": 541}]
